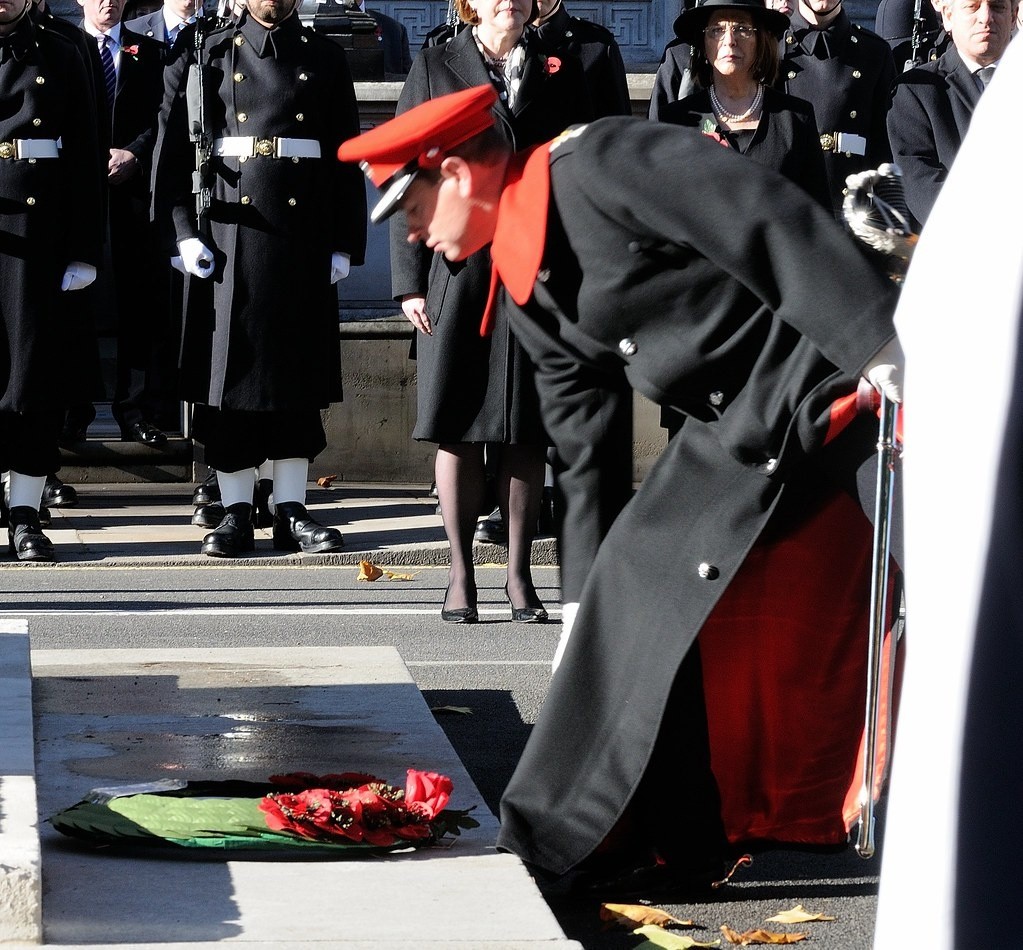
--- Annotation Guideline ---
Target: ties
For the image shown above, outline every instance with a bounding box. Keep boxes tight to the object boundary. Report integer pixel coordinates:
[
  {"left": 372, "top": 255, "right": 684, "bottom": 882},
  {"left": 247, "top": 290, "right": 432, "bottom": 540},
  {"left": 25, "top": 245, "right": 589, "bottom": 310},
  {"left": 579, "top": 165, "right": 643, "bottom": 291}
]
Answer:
[{"left": 100, "top": 34, "right": 116, "bottom": 143}]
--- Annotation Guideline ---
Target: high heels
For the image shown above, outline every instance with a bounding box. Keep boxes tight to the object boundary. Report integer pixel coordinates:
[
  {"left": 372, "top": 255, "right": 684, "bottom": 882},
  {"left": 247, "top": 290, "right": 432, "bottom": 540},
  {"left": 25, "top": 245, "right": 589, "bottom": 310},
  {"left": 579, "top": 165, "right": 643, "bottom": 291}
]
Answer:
[
  {"left": 504, "top": 580, "right": 549, "bottom": 622},
  {"left": 441, "top": 583, "right": 479, "bottom": 622}
]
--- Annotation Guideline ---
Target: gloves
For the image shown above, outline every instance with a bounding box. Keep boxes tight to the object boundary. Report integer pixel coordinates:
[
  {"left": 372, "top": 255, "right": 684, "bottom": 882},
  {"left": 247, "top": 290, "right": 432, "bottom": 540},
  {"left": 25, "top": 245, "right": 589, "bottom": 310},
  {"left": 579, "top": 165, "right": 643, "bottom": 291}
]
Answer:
[
  {"left": 176, "top": 238, "right": 216, "bottom": 278},
  {"left": 62, "top": 261, "right": 97, "bottom": 291},
  {"left": 331, "top": 252, "right": 351, "bottom": 284},
  {"left": 550, "top": 602, "right": 581, "bottom": 676},
  {"left": 861, "top": 333, "right": 907, "bottom": 404}
]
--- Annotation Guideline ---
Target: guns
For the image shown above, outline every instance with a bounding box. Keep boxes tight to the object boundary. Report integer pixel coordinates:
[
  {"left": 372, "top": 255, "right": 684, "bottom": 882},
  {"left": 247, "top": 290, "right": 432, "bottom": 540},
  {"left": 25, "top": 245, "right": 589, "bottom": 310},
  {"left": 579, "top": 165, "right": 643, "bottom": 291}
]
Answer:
[
  {"left": 445, "top": 0, "right": 460, "bottom": 43},
  {"left": 184, "top": 0, "right": 212, "bottom": 269},
  {"left": 902, "top": 0, "right": 927, "bottom": 73},
  {"left": 678, "top": 0, "right": 704, "bottom": 101}
]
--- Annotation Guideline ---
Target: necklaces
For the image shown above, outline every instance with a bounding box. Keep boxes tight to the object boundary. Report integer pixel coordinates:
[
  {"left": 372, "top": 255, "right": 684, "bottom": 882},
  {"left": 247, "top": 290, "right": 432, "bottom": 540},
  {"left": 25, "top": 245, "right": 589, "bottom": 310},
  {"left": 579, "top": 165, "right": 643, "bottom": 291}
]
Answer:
[{"left": 705, "top": 80, "right": 764, "bottom": 125}]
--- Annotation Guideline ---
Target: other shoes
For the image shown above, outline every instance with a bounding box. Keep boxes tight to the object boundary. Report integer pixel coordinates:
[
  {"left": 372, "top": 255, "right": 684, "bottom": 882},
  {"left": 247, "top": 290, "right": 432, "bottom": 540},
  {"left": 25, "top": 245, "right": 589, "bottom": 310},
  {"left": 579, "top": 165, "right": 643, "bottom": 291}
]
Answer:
[
  {"left": 435, "top": 504, "right": 443, "bottom": 515},
  {"left": 428, "top": 481, "right": 438, "bottom": 497}
]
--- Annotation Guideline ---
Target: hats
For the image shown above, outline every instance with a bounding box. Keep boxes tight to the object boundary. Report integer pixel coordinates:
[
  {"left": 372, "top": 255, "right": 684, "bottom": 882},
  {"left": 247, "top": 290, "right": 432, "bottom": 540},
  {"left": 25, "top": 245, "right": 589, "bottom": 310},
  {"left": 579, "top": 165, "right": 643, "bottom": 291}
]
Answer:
[
  {"left": 337, "top": 83, "right": 498, "bottom": 225},
  {"left": 672, "top": 0, "right": 792, "bottom": 47}
]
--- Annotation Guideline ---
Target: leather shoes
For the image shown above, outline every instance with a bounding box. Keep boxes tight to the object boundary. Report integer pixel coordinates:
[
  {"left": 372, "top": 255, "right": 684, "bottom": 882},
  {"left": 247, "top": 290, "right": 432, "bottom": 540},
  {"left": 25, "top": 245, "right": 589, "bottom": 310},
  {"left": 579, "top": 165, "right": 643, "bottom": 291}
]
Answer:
[
  {"left": 40, "top": 482, "right": 77, "bottom": 507},
  {"left": 2, "top": 504, "right": 51, "bottom": 525},
  {"left": 258, "top": 493, "right": 276, "bottom": 521},
  {"left": 121, "top": 422, "right": 167, "bottom": 444},
  {"left": 7, "top": 524, "right": 52, "bottom": 559},
  {"left": 191, "top": 500, "right": 228, "bottom": 527},
  {"left": 200, "top": 512, "right": 255, "bottom": 557},
  {"left": 273, "top": 508, "right": 344, "bottom": 553},
  {"left": 475, "top": 506, "right": 505, "bottom": 541},
  {"left": 193, "top": 474, "right": 221, "bottom": 506}
]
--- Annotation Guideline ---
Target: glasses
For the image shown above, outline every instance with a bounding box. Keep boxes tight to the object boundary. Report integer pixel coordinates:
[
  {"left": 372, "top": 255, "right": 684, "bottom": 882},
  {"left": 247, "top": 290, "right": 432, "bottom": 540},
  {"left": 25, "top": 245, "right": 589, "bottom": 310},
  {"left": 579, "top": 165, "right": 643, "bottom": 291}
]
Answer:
[{"left": 701, "top": 25, "right": 758, "bottom": 41}]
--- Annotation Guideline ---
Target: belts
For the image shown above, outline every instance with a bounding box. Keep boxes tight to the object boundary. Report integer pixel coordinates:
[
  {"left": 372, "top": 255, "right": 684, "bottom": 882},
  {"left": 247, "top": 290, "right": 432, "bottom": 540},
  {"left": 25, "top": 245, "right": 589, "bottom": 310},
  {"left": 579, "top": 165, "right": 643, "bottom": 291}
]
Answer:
[
  {"left": 212, "top": 136, "right": 322, "bottom": 158},
  {"left": 18, "top": 139, "right": 59, "bottom": 159},
  {"left": 838, "top": 132, "right": 867, "bottom": 156}
]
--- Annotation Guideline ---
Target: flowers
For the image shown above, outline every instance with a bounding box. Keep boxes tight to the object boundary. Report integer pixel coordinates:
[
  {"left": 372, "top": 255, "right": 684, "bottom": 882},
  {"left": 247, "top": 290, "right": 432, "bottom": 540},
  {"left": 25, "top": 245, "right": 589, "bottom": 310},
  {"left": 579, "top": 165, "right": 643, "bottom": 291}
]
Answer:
[
  {"left": 256, "top": 766, "right": 457, "bottom": 852},
  {"left": 376, "top": 26, "right": 383, "bottom": 41},
  {"left": 536, "top": 52, "right": 562, "bottom": 83},
  {"left": 699, "top": 119, "right": 729, "bottom": 151},
  {"left": 116, "top": 44, "right": 141, "bottom": 62}
]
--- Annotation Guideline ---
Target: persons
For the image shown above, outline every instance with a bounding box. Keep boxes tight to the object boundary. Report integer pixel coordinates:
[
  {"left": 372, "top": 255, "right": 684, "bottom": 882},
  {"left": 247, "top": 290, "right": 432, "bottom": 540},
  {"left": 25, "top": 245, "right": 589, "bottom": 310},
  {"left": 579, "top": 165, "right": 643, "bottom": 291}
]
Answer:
[
  {"left": 296, "top": 0, "right": 413, "bottom": 83},
  {"left": 0, "top": 0, "right": 111, "bottom": 564},
  {"left": 388, "top": 0, "right": 631, "bottom": 626},
  {"left": 335, "top": 79, "right": 904, "bottom": 904},
  {"left": 124, "top": 0, "right": 217, "bottom": 50},
  {"left": 148, "top": 0, "right": 368, "bottom": 557},
  {"left": 53, "top": 0, "right": 170, "bottom": 446},
  {"left": 647, "top": 0, "right": 1023, "bottom": 950}
]
